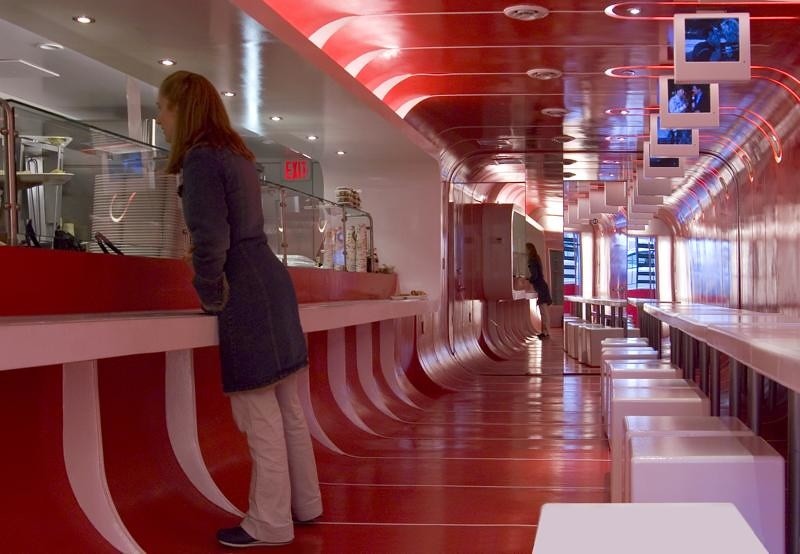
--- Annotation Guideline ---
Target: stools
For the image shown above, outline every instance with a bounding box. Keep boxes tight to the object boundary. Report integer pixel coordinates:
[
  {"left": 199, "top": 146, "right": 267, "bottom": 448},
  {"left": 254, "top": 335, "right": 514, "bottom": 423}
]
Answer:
[
  {"left": 531, "top": 338, "right": 786, "bottom": 554},
  {"left": 567, "top": 321, "right": 594, "bottom": 359},
  {"left": 586, "top": 327, "right": 624, "bottom": 365},
  {"left": 577, "top": 326, "right": 610, "bottom": 363},
  {"left": 564, "top": 318, "right": 588, "bottom": 353}
]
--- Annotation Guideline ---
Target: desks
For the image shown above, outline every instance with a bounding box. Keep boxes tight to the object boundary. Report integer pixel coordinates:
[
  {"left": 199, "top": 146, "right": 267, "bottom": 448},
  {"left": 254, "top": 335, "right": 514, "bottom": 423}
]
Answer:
[
  {"left": 642, "top": 304, "right": 800, "bottom": 554},
  {"left": 628, "top": 298, "right": 680, "bottom": 341},
  {"left": 601, "top": 299, "right": 628, "bottom": 327},
  {"left": 583, "top": 298, "right": 600, "bottom": 326},
  {"left": 565, "top": 295, "right": 584, "bottom": 317}
]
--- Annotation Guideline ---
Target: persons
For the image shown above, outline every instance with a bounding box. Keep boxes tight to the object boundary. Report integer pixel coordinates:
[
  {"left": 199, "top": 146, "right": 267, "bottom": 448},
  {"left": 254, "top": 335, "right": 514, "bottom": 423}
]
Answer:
[
  {"left": 156, "top": 70, "right": 322, "bottom": 548},
  {"left": 668, "top": 85, "right": 705, "bottom": 113},
  {"left": 516, "top": 243, "right": 552, "bottom": 339},
  {"left": 692, "top": 17, "right": 739, "bottom": 61}
]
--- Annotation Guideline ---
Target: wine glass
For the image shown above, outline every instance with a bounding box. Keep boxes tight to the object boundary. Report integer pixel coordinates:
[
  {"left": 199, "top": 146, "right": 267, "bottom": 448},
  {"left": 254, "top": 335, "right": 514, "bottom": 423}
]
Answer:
[{"left": 47, "top": 137, "right": 72, "bottom": 173}]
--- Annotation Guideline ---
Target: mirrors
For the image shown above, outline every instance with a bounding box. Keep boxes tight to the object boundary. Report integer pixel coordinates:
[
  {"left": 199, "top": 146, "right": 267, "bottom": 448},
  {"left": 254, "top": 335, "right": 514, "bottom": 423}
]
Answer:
[{"left": 446, "top": 154, "right": 738, "bottom": 375}]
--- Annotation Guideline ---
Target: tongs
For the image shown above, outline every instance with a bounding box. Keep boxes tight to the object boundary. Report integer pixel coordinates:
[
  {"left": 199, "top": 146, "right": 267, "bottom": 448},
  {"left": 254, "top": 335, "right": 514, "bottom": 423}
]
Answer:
[{"left": 94, "top": 232, "right": 125, "bottom": 256}]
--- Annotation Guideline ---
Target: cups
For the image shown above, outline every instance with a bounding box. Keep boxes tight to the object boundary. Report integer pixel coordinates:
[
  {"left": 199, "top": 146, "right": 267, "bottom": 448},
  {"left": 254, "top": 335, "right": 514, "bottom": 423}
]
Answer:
[
  {"left": 324, "top": 226, "right": 344, "bottom": 270},
  {"left": 346, "top": 225, "right": 357, "bottom": 272},
  {"left": 355, "top": 223, "right": 367, "bottom": 272}
]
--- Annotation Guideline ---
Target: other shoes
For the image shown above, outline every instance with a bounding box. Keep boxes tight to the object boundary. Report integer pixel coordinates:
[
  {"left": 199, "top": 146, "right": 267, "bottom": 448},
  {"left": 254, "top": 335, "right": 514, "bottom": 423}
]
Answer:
[
  {"left": 537, "top": 333, "right": 549, "bottom": 337},
  {"left": 217, "top": 526, "right": 293, "bottom": 547}
]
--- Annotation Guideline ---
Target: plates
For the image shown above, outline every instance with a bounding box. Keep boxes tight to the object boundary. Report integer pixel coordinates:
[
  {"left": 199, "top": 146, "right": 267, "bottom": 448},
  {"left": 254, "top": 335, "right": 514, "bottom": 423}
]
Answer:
[
  {"left": 406, "top": 296, "right": 427, "bottom": 299},
  {"left": 390, "top": 296, "right": 406, "bottom": 299},
  {"left": 90, "top": 173, "right": 192, "bottom": 258}
]
